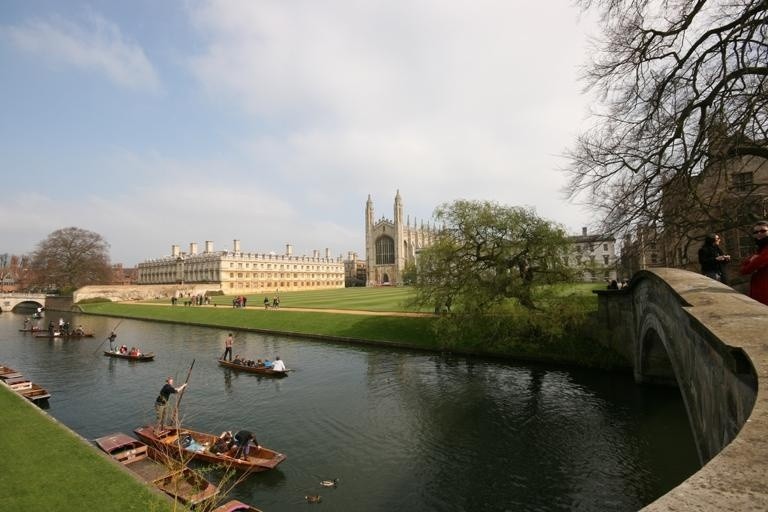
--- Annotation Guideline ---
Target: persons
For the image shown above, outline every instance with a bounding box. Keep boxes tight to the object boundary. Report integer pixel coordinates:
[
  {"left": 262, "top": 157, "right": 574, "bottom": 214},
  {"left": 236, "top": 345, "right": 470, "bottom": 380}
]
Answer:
[
  {"left": 210, "top": 429, "right": 235, "bottom": 453},
  {"left": 738, "top": 221, "right": 768, "bottom": 307},
  {"left": 152, "top": 375, "right": 188, "bottom": 433},
  {"left": 698, "top": 232, "right": 731, "bottom": 284},
  {"left": 221, "top": 331, "right": 290, "bottom": 376},
  {"left": 162, "top": 290, "right": 286, "bottom": 307},
  {"left": 107, "top": 332, "right": 145, "bottom": 359},
  {"left": 234, "top": 429, "right": 261, "bottom": 460},
  {"left": 23, "top": 307, "right": 86, "bottom": 340}
]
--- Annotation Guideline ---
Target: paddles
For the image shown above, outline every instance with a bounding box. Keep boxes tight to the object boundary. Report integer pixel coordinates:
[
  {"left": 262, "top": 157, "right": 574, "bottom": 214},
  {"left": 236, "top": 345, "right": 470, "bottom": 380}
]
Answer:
[
  {"left": 220, "top": 332, "right": 239, "bottom": 360},
  {"left": 172, "top": 360, "right": 194, "bottom": 420}
]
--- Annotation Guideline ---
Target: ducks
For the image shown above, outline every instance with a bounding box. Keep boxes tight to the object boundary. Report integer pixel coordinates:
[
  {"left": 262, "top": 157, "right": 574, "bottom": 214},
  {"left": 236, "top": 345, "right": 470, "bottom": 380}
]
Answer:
[
  {"left": 304, "top": 495, "right": 323, "bottom": 502},
  {"left": 320, "top": 477, "right": 341, "bottom": 487}
]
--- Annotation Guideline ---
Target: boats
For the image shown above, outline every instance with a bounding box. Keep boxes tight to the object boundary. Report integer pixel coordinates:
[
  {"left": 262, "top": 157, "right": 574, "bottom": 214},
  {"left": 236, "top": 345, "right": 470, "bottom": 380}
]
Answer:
[
  {"left": 1, "top": 365, "right": 52, "bottom": 403},
  {"left": 17, "top": 326, "right": 96, "bottom": 338},
  {"left": 102, "top": 350, "right": 157, "bottom": 361},
  {"left": 218, "top": 356, "right": 296, "bottom": 375},
  {"left": 95, "top": 431, "right": 267, "bottom": 512},
  {"left": 132, "top": 421, "right": 287, "bottom": 479}
]
